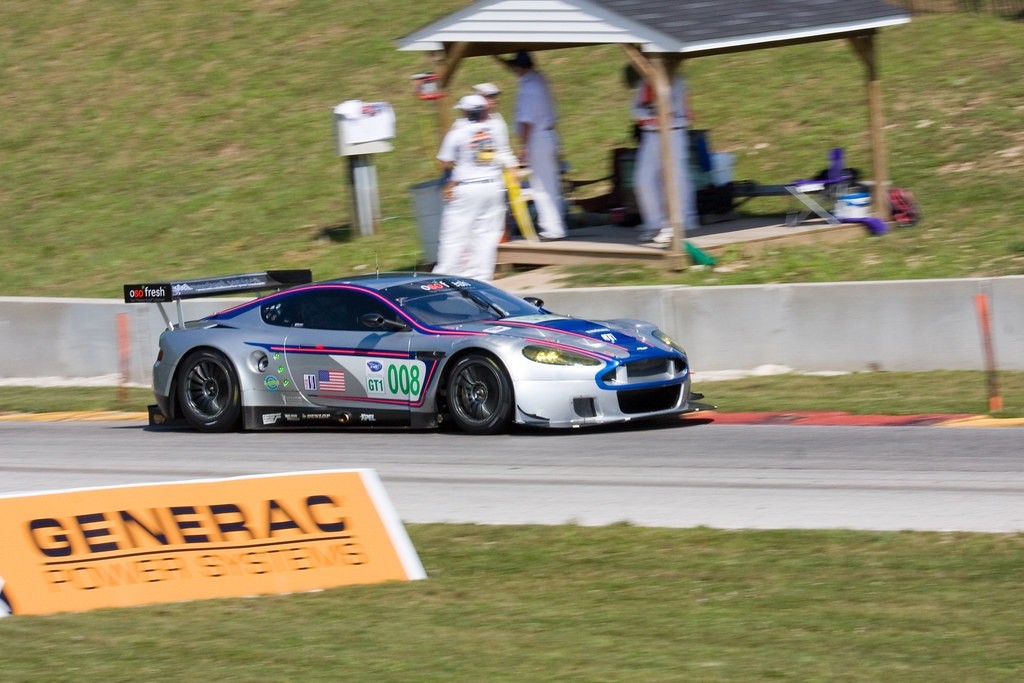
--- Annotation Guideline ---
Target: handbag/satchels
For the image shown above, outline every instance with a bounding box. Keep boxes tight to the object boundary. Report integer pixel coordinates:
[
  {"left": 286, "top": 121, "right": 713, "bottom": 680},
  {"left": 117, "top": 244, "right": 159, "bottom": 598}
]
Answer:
[
  {"left": 890, "top": 188, "right": 921, "bottom": 225},
  {"left": 834, "top": 192, "right": 872, "bottom": 219}
]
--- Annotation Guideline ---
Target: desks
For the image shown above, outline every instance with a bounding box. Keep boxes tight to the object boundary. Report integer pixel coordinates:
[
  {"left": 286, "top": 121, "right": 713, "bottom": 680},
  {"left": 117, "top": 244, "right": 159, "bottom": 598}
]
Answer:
[{"left": 728, "top": 179, "right": 847, "bottom": 228}]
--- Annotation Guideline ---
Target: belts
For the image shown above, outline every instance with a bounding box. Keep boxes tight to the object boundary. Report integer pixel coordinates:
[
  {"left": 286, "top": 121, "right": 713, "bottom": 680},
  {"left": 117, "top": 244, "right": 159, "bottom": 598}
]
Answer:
[
  {"left": 644, "top": 126, "right": 686, "bottom": 132},
  {"left": 544, "top": 127, "right": 555, "bottom": 131},
  {"left": 455, "top": 179, "right": 494, "bottom": 184}
]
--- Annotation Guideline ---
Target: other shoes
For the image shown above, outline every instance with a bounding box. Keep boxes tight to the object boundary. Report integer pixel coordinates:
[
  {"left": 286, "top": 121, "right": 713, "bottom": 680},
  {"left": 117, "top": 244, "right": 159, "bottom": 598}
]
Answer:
[
  {"left": 654, "top": 228, "right": 674, "bottom": 243},
  {"left": 537, "top": 233, "right": 565, "bottom": 241},
  {"left": 636, "top": 231, "right": 657, "bottom": 241}
]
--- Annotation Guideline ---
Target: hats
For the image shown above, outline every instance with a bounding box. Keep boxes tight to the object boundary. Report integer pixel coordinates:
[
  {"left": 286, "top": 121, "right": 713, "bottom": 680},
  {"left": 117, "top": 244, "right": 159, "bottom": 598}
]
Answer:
[
  {"left": 506, "top": 51, "right": 534, "bottom": 69},
  {"left": 453, "top": 95, "right": 490, "bottom": 111},
  {"left": 472, "top": 83, "right": 501, "bottom": 96}
]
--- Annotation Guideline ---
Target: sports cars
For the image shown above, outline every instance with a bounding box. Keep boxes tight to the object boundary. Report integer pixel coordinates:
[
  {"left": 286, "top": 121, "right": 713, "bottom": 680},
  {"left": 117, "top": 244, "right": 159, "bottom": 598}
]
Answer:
[{"left": 121, "top": 266, "right": 717, "bottom": 437}]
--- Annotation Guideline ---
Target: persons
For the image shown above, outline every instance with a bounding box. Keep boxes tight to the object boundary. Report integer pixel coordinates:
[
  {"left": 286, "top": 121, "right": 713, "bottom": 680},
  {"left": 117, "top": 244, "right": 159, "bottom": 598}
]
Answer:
[
  {"left": 493, "top": 50, "right": 569, "bottom": 242},
  {"left": 620, "top": 52, "right": 698, "bottom": 244},
  {"left": 433, "top": 82, "right": 522, "bottom": 282}
]
